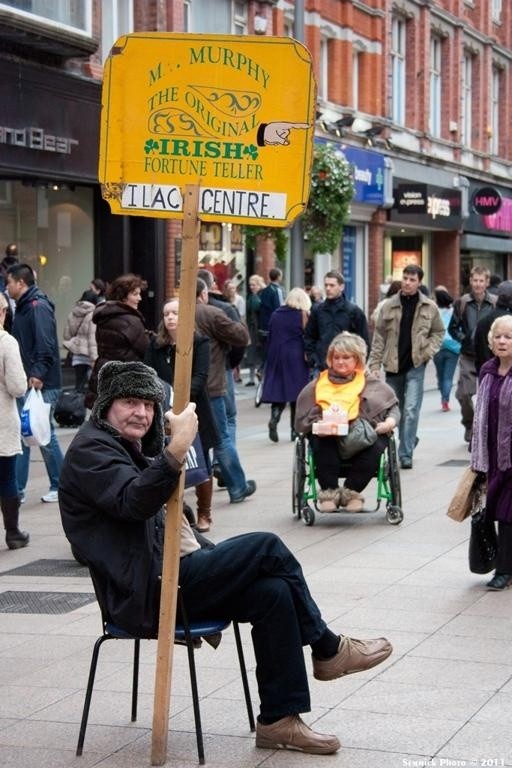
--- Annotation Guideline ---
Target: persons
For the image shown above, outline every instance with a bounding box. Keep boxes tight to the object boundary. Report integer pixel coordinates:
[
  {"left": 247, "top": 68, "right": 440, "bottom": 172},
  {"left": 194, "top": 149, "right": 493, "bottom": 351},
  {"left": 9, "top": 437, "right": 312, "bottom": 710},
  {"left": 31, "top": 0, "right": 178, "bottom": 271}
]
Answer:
[
  {"left": 447, "top": 265, "right": 512, "bottom": 590},
  {"left": 64, "top": 270, "right": 256, "bottom": 533},
  {"left": 57, "top": 359, "right": 393, "bottom": 755},
  {"left": 0, "top": 263, "right": 64, "bottom": 548},
  {"left": 248, "top": 263, "right": 459, "bottom": 512},
  {"left": 0, "top": 242, "right": 20, "bottom": 291}
]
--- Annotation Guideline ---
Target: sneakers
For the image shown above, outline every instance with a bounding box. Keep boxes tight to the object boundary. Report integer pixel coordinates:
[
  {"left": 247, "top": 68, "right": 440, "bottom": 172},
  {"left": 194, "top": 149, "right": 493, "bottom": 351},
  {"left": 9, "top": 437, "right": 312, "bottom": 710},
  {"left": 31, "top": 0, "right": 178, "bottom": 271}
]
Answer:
[
  {"left": 401, "top": 456, "right": 411, "bottom": 469},
  {"left": 318, "top": 487, "right": 364, "bottom": 513},
  {"left": 465, "top": 430, "right": 472, "bottom": 452},
  {"left": 230, "top": 480, "right": 255, "bottom": 503},
  {"left": 41, "top": 491, "right": 58, "bottom": 502}
]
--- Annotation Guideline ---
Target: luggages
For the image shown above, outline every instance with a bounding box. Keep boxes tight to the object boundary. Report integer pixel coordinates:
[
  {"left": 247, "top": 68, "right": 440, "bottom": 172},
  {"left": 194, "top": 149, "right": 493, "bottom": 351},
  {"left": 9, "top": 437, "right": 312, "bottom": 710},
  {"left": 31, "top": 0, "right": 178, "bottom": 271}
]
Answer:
[{"left": 54, "top": 390, "right": 85, "bottom": 428}]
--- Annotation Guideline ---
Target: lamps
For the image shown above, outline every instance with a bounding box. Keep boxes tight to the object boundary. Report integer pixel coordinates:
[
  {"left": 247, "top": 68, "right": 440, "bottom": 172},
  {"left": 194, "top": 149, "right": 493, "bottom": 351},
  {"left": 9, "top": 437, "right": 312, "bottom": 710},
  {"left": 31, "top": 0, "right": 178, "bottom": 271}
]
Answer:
[{"left": 316, "top": 110, "right": 384, "bottom": 149}]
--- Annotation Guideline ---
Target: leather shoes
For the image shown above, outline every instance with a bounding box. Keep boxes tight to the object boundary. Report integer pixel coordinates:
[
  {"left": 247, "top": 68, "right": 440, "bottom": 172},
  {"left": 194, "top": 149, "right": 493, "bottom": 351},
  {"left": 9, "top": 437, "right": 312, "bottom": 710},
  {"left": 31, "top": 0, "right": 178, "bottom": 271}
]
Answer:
[
  {"left": 256, "top": 713, "right": 340, "bottom": 753},
  {"left": 487, "top": 574, "right": 512, "bottom": 590},
  {"left": 312, "top": 634, "right": 392, "bottom": 680}
]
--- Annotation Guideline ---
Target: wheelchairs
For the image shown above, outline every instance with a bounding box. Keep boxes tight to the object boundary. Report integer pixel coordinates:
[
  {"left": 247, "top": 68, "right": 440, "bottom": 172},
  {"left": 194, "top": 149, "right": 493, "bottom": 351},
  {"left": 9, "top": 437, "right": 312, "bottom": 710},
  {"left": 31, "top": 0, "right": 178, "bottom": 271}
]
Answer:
[{"left": 288, "top": 423, "right": 410, "bottom": 525}]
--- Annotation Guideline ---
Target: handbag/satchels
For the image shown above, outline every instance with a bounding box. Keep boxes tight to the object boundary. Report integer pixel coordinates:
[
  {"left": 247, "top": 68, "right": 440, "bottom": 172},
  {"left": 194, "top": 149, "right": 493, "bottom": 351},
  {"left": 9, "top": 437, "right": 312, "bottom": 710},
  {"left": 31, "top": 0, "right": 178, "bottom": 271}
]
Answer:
[
  {"left": 166, "top": 431, "right": 210, "bottom": 489},
  {"left": 447, "top": 466, "right": 496, "bottom": 573},
  {"left": 337, "top": 417, "right": 377, "bottom": 460}
]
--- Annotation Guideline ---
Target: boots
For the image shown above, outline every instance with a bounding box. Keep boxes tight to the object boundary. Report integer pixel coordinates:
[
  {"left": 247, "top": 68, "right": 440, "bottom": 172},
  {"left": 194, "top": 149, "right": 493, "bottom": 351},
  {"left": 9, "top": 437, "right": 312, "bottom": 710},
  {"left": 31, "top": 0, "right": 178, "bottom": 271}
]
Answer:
[
  {"left": 0, "top": 496, "right": 28, "bottom": 549},
  {"left": 269, "top": 406, "right": 281, "bottom": 441},
  {"left": 196, "top": 476, "right": 212, "bottom": 531}
]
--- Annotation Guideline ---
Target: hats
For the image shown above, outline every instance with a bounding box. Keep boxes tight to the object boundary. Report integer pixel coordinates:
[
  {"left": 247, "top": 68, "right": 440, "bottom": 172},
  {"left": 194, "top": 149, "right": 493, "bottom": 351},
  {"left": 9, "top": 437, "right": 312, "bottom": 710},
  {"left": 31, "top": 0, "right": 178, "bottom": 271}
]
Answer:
[{"left": 92, "top": 361, "right": 165, "bottom": 457}]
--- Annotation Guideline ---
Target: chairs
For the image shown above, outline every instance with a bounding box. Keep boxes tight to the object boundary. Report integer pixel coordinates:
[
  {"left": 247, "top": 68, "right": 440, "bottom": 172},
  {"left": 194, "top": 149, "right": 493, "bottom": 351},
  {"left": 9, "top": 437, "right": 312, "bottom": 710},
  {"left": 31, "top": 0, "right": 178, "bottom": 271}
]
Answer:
[{"left": 70, "top": 545, "right": 256, "bottom": 765}]
